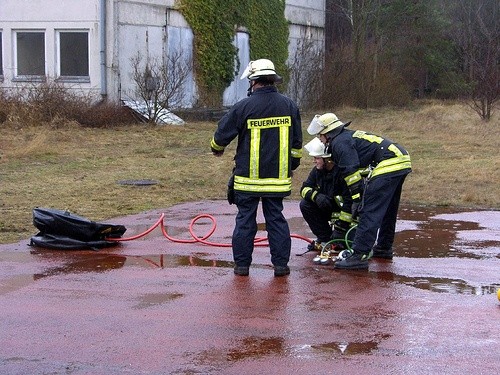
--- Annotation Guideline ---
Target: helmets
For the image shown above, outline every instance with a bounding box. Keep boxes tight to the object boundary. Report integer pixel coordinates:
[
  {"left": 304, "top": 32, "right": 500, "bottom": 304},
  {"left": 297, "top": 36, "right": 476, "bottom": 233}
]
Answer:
[
  {"left": 307, "top": 113, "right": 352, "bottom": 140},
  {"left": 240, "top": 59, "right": 283, "bottom": 84},
  {"left": 304, "top": 137, "right": 331, "bottom": 157}
]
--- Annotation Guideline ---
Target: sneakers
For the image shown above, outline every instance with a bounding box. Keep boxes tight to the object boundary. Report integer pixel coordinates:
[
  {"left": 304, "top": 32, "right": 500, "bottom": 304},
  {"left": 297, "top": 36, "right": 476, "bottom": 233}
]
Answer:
[
  {"left": 373, "top": 249, "right": 393, "bottom": 259},
  {"left": 274, "top": 265, "right": 291, "bottom": 277},
  {"left": 335, "top": 254, "right": 369, "bottom": 269},
  {"left": 308, "top": 239, "right": 331, "bottom": 251},
  {"left": 234, "top": 265, "right": 249, "bottom": 276}
]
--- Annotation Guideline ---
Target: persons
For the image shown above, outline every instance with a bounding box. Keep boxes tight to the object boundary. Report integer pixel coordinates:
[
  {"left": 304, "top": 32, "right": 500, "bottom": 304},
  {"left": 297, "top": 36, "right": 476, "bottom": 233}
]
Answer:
[
  {"left": 309, "top": 113, "right": 412, "bottom": 269},
  {"left": 297, "top": 136, "right": 351, "bottom": 256},
  {"left": 211, "top": 59, "right": 302, "bottom": 277}
]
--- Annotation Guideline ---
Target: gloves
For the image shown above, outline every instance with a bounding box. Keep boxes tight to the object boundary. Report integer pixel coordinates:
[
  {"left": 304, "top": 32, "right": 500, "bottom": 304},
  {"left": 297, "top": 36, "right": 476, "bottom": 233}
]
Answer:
[
  {"left": 314, "top": 193, "right": 332, "bottom": 209},
  {"left": 352, "top": 202, "right": 361, "bottom": 220}
]
